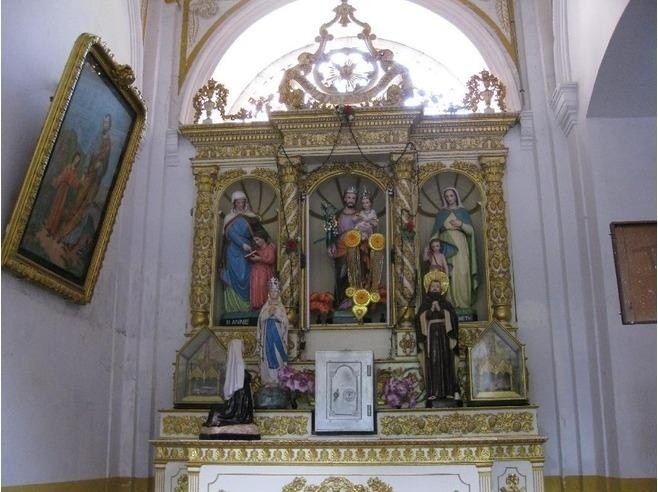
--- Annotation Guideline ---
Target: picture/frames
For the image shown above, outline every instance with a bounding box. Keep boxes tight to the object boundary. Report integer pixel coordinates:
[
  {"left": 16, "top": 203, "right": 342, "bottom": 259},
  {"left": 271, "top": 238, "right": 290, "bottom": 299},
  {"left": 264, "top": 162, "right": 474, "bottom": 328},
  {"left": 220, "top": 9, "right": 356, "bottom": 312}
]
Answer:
[
  {"left": 0, "top": 30, "right": 149, "bottom": 306},
  {"left": 610, "top": 219, "right": 657, "bottom": 325}
]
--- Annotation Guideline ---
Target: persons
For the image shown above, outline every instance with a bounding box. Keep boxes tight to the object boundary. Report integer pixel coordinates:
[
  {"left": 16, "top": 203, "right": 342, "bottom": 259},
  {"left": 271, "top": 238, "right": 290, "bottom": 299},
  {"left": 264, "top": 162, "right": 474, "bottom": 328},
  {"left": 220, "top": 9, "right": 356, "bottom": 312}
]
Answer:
[{"left": 43, "top": 113, "right": 112, "bottom": 263}]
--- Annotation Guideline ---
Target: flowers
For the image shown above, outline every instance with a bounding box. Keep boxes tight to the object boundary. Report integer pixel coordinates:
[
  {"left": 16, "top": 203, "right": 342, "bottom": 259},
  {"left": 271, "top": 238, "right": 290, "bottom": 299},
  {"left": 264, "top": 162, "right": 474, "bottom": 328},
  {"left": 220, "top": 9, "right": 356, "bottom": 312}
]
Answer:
[
  {"left": 381, "top": 374, "right": 418, "bottom": 409},
  {"left": 278, "top": 365, "right": 314, "bottom": 396}
]
